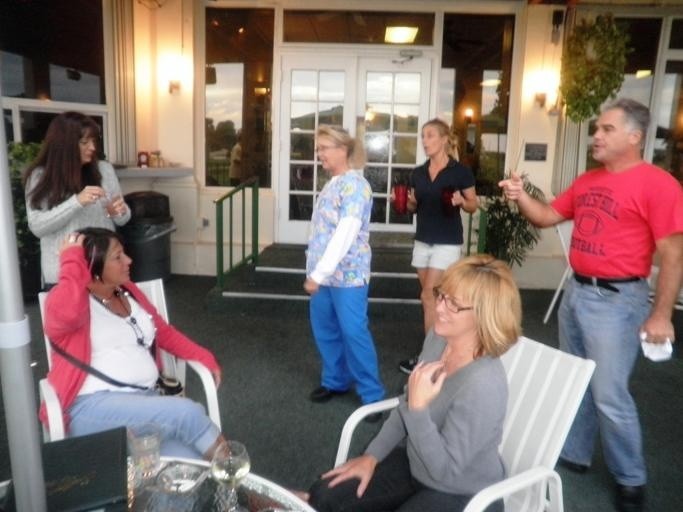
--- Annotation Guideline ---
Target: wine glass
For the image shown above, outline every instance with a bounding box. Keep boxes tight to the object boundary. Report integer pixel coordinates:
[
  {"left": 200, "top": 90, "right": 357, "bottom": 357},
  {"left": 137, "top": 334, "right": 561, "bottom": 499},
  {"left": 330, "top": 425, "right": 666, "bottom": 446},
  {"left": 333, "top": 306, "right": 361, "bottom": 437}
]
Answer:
[{"left": 208, "top": 441, "right": 251, "bottom": 511}]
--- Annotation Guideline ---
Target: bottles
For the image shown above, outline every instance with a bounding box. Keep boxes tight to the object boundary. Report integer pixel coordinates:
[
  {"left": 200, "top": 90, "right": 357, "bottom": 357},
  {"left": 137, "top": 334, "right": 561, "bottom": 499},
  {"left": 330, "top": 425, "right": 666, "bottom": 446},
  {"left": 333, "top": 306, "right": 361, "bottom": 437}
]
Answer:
[{"left": 136, "top": 150, "right": 164, "bottom": 169}]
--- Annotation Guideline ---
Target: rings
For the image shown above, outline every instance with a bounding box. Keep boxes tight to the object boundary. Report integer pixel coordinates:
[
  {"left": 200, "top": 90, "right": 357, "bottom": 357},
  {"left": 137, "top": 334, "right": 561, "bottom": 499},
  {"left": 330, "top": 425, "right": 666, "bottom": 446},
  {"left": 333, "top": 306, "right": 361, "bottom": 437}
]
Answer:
[{"left": 68, "top": 239, "right": 74, "bottom": 243}]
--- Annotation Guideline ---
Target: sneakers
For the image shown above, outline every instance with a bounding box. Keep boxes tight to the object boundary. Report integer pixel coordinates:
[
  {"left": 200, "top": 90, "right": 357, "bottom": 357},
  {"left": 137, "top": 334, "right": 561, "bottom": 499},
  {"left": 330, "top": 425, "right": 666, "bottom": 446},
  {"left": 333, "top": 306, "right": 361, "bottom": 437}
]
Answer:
[{"left": 398, "top": 356, "right": 418, "bottom": 374}]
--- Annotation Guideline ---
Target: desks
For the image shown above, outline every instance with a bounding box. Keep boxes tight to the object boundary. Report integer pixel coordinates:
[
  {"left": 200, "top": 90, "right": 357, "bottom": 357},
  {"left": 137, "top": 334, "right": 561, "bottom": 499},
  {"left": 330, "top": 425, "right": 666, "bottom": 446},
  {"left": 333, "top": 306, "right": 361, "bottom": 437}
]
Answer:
[{"left": 1, "top": 455, "right": 320, "bottom": 509}]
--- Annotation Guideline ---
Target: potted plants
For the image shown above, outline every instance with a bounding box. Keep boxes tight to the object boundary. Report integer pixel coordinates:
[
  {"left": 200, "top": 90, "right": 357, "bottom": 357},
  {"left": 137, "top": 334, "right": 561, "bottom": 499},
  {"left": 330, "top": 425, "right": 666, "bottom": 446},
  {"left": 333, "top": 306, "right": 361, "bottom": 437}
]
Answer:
[{"left": 6, "top": 143, "right": 48, "bottom": 305}]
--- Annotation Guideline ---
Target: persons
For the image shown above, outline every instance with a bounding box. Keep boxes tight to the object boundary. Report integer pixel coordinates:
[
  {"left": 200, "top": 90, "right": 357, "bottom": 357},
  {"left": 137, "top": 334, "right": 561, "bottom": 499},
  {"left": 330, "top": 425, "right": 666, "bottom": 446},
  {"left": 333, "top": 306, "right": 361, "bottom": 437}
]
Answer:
[
  {"left": 308, "top": 253, "right": 523, "bottom": 510},
  {"left": 37, "top": 227, "right": 231, "bottom": 471},
  {"left": 496, "top": 97, "right": 683, "bottom": 510},
  {"left": 302, "top": 125, "right": 385, "bottom": 422},
  {"left": 227, "top": 132, "right": 243, "bottom": 186},
  {"left": 388, "top": 117, "right": 477, "bottom": 373},
  {"left": 20, "top": 111, "right": 132, "bottom": 291}
]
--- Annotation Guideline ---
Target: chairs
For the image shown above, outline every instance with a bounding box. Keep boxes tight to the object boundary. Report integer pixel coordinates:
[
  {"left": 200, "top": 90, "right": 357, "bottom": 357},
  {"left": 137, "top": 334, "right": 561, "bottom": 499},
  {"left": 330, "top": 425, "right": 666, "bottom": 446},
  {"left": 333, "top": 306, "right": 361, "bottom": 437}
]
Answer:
[
  {"left": 36, "top": 276, "right": 221, "bottom": 442},
  {"left": 541, "top": 193, "right": 575, "bottom": 328},
  {"left": 332, "top": 317, "right": 596, "bottom": 512}
]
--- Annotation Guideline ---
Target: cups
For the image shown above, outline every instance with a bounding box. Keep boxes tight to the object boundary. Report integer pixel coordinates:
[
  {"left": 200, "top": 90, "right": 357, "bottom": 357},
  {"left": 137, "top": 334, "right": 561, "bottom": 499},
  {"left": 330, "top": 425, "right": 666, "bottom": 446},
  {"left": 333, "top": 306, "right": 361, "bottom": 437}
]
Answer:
[
  {"left": 126, "top": 421, "right": 159, "bottom": 476},
  {"left": 96, "top": 193, "right": 124, "bottom": 220},
  {"left": 393, "top": 184, "right": 407, "bottom": 214}
]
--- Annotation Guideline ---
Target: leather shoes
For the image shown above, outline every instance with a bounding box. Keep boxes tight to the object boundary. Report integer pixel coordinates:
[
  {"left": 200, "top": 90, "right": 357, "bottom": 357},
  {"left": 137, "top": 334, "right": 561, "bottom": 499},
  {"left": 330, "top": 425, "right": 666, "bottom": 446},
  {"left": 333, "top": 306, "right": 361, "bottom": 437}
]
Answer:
[
  {"left": 309, "top": 386, "right": 349, "bottom": 403},
  {"left": 613, "top": 485, "right": 650, "bottom": 512},
  {"left": 559, "top": 457, "right": 588, "bottom": 472},
  {"left": 365, "top": 400, "right": 383, "bottom": 423}
]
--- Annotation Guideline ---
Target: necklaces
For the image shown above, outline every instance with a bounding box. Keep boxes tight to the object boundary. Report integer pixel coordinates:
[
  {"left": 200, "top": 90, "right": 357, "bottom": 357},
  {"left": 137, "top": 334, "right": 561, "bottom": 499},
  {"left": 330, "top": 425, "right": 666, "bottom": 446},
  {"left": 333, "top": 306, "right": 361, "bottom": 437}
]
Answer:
[{"left": 85, "top": 283, "right": 145, "bottom": 347}]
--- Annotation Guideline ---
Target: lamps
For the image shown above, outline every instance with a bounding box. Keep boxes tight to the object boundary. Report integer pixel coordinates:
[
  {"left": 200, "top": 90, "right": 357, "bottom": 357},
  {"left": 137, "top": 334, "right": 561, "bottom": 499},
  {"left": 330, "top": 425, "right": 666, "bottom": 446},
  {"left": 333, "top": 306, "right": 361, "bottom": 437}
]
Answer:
[
  {"left": 536, "top": 63, "right": 558, "bottom": 118},
  {"left": 161, "top": 52, "right": 186, "bottom": 96}
]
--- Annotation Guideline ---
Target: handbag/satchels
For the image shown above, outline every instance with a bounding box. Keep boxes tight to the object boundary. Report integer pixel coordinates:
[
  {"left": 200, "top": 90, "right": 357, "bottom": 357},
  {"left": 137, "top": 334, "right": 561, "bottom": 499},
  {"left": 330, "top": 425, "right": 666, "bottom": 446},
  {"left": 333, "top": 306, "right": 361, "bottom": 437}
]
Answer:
[{"left": 158, "top": 375, "right": 184, "bottom": 397}]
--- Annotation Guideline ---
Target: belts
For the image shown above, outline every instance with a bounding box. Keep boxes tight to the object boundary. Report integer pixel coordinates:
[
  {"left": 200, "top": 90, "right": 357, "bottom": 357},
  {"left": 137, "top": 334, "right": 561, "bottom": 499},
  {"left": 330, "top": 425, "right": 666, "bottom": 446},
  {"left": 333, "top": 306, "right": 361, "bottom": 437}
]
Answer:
[{"left": 575, "top": 273, "right": 641, "bottom": 293}]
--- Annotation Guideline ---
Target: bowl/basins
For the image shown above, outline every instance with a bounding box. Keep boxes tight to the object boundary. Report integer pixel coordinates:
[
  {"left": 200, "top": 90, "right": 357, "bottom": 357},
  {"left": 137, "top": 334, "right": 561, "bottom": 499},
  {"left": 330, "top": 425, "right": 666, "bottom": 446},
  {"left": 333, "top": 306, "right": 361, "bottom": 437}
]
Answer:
[{"left": 157, "top": 460, "right": 208, "bottom": 496}]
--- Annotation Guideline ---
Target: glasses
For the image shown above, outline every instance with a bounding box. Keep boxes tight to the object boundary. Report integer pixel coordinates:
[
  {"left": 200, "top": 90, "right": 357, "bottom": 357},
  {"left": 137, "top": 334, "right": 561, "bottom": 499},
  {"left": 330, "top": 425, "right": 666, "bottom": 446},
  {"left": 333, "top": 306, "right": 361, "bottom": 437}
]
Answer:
[{"left": 432, "top": 285, "right": 474, "bottom": 314}]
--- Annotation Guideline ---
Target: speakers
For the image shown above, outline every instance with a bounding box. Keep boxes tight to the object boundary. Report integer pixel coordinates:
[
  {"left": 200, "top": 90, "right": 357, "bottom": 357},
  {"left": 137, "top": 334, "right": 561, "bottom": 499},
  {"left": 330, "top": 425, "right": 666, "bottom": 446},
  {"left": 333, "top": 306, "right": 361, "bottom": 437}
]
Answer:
[{"left": 441, "top": 11, "right": 505, "bottom": 70}]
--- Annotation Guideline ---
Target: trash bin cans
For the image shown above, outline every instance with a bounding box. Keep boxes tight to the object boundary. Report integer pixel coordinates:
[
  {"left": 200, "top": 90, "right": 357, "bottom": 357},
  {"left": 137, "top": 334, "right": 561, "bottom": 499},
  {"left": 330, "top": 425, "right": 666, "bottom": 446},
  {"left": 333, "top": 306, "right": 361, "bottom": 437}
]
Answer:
[{"left": 115, "top": 190, "right": 176, "bottom": 283}]
